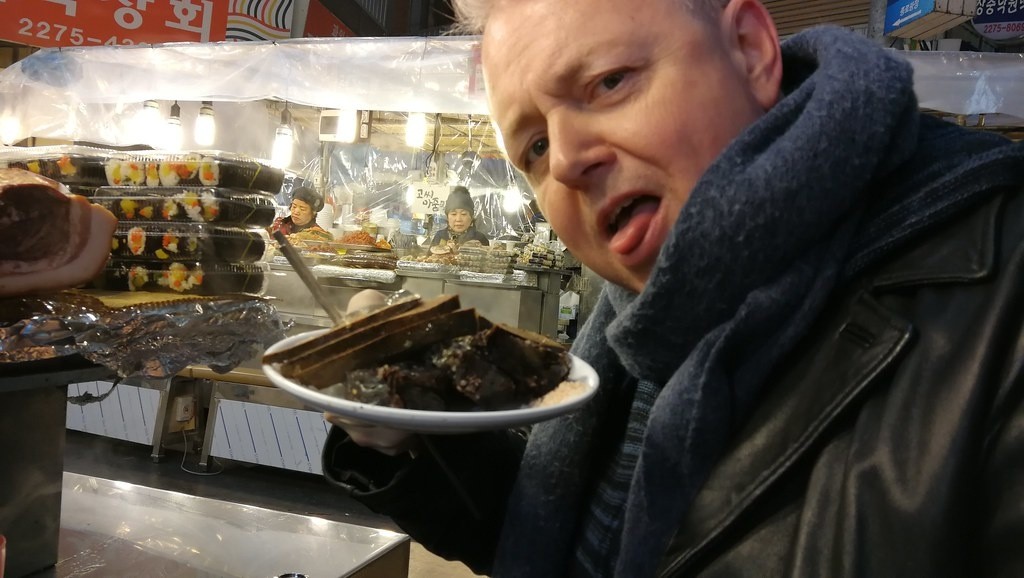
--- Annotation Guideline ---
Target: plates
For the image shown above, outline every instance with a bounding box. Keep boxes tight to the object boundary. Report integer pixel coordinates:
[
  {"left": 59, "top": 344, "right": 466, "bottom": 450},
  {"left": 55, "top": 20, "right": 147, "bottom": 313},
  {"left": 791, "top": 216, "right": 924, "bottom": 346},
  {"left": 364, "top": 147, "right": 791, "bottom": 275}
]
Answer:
[{"left": 261, "top": 327, "right": 601, "bottom": 432}]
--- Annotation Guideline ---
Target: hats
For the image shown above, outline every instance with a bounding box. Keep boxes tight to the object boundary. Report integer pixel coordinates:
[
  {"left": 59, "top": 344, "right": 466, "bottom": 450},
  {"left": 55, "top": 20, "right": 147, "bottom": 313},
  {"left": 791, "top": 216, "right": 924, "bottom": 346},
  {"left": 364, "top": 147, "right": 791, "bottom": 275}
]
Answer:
[
  {"left": 445, "top": 186, "right": 474, "bottom": 216},
  {"left": 292, "top": 187, "right": 324, "bottom": 211}
]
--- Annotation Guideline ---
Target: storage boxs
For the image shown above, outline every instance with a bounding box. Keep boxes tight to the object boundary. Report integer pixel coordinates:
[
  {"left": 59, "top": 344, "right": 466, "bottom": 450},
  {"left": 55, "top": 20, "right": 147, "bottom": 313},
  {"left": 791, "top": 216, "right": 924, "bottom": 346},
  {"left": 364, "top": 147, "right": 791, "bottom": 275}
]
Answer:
[{"left": 0, "top": 146, "right": 286, "bottom": 297}]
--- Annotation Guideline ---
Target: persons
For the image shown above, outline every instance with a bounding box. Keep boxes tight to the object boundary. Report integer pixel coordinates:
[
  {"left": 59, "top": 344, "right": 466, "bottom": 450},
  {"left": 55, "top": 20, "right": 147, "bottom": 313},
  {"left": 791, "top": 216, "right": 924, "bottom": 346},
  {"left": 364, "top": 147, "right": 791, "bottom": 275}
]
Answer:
[
  {"left": 427, "top": 186, "right": 489, "bottom": 258},
  {"left": 508, "top": 199, "right": 546, "bottom": 241},
  {"left": 269, "top": 187, "right": 324, "bottom": 239},
  {"left": 321, "top": 1, "right": 1024, "bottom": 578}
]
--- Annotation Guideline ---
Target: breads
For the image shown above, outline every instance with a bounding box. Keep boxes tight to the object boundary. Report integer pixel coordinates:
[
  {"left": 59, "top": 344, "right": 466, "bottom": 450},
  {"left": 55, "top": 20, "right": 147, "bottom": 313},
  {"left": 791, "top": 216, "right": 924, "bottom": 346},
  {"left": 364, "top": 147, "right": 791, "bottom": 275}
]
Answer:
[{"left": 262, "top": 295, "right": 573, "bottom": 412}]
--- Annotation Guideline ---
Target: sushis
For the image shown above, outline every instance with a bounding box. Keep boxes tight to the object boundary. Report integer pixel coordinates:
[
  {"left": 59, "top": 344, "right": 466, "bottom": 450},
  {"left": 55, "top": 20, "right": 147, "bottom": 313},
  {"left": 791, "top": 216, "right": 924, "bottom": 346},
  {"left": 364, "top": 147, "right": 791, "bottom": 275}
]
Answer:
[{"left": 6, "top": 152, "right": 286, "bottom": 297}]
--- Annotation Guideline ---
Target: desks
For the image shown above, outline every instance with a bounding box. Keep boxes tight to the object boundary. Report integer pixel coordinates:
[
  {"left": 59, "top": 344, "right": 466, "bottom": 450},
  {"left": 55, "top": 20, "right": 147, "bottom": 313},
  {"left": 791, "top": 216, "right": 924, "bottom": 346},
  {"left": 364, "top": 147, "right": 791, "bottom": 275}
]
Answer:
[{"left": 57, "top": 472, "right": 413, "bottom": 578}]
